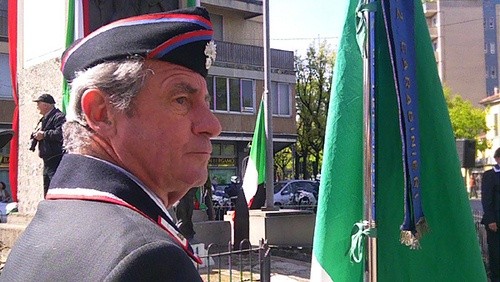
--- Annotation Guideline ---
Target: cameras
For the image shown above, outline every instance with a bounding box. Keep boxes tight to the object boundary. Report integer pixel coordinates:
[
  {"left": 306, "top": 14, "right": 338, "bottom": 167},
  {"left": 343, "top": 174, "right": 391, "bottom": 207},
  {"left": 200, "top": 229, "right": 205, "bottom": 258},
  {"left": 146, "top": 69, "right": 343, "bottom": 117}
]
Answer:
[{"left": 29, "top": 132, "right": 38, "bottom": 152}]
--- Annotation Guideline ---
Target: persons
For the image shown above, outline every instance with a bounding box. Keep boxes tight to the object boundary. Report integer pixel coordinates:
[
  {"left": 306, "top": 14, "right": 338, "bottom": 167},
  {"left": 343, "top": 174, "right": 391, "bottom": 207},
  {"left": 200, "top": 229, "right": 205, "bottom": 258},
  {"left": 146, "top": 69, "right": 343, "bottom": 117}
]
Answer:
[
  {"left": 0, "top": 182, "right": 10, "bottom": 203},
  {"left": 480, "top": 147, "right": 500, "bottom": 282},
  {"left": 0, "top": 7, "right": 221, "bottom": 282},
  {"left": 30, "top": 94, "right": 66, "bottom": 199},
  {"left": 211, "top": 173, "right": 242, "bottom": 210}
]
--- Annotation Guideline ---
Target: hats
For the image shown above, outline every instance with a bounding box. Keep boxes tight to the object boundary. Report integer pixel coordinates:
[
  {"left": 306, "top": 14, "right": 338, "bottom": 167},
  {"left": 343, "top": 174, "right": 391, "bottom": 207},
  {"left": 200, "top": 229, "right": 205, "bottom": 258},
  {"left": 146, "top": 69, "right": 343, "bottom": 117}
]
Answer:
[
  {"left": 32, "top": 93, "right": 56, "bottom": 104},
  {"left": 58, "top": 4, "right": 218, "bottom": 77}
]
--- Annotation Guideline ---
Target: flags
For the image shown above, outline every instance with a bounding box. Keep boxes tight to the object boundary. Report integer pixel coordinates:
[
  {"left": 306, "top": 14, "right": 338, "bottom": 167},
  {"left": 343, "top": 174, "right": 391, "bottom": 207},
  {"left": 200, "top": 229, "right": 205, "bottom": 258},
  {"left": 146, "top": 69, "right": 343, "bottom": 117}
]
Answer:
[
  {"left": 242, "top": 94, "right": 267, "bottom": 207},
  {"left": 62, "top": 0, "right": 90, "bottom": 117},
  {"left": 310, "top": 1, "right": 489, "bottom": 281}
]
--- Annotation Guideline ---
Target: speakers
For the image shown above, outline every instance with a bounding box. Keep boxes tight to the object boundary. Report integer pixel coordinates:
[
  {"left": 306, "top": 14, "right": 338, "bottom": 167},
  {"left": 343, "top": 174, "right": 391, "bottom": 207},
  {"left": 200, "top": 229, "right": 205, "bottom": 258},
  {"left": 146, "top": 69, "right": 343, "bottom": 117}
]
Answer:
[{"left": 456, "top": 139, "right": 476, "bottom": 169}]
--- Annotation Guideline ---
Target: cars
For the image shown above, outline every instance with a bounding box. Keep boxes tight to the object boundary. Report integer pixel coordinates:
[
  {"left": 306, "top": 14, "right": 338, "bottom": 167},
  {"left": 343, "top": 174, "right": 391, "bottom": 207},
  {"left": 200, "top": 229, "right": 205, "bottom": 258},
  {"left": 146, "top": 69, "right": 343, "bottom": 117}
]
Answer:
[{"left": 273, "top": 180, "right": 320, "bottom": 210}]
